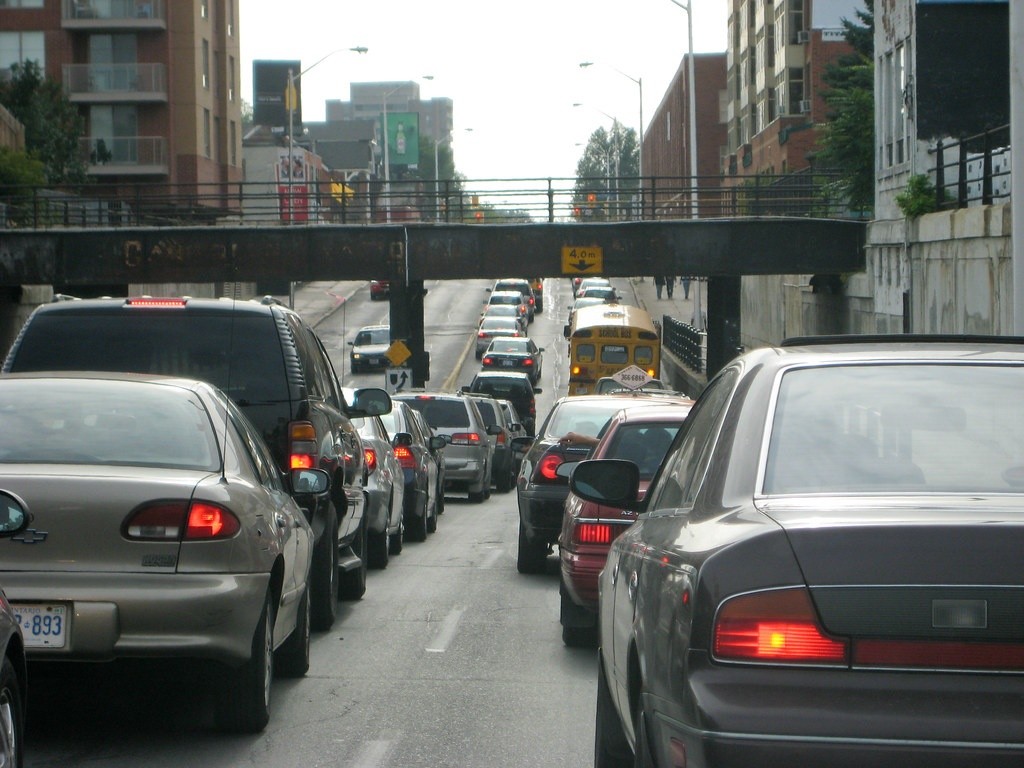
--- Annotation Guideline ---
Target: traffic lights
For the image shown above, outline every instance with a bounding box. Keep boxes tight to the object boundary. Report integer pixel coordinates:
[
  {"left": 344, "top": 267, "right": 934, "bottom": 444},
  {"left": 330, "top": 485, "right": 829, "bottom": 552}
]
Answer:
[
  {"left": 574, "top": 205, "right": 581, "bottom": 215},
  {"left": 476, "top": 209, "right": 484, "bottom": 225},
  {"left": 587, "top": 193, "right": 596, "bottom": 206}
]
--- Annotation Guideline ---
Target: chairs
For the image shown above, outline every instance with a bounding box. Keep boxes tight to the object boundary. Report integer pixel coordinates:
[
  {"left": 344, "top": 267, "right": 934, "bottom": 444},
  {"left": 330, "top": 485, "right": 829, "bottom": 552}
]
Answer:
[{"left": 614, "top": 429, "right": 673, "bottom": 471}]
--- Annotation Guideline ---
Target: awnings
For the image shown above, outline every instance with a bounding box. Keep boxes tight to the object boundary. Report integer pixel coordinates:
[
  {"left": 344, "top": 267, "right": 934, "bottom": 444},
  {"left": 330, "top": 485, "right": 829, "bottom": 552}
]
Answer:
[{"left": 332, "top": 179, "right": 354, "bottom": 206}]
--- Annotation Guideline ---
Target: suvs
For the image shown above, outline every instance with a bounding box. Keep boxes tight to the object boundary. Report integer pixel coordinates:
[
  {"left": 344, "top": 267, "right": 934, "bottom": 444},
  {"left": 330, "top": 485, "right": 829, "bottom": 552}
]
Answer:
[{"left": 0, "top": 292, "right": 390, "bottom": 633}]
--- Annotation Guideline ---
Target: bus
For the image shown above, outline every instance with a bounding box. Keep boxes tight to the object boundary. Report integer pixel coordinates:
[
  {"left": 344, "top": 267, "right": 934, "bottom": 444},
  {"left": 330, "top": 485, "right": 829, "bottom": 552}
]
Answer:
[{"left": 563, "top": 304, "right": 662, "bottom": 397}]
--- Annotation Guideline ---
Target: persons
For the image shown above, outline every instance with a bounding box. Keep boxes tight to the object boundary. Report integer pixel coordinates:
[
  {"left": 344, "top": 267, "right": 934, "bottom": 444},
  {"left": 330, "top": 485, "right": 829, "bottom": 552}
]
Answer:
[
  {"left": 666, "top": 276, "right": 676, "bottom": 299},
  {"left": 680, "top": 277, "right": 691, "bottom": 299},
  {"left": 653, "top": 277, "right": 665, "bottom": 298}
]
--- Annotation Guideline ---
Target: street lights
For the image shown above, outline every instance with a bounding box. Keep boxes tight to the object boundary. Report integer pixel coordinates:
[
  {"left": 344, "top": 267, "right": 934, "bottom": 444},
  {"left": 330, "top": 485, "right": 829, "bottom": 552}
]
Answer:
[
  {"left": 574, "top": 143, "right": 609, "bottom": 221},
  {"left": 383, "top": 75, "right": 434, "bottom": 222},
  {"left": 289, "top": 46, "right": 367, "bottom": 222},
  {"left": 580, "top": 61, "right": 643, "bottom": 220},
  {"left": 434, "top": 126, "right": 473, "bottom": 222},
  {"left": 573, "top": 102, "right": 620, "bottom": 221}
]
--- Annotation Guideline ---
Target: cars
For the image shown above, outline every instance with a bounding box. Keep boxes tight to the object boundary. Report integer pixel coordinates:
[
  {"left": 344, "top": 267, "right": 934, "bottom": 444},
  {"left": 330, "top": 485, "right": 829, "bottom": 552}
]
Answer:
[
  {"left": 555, "top": 401, "right": 706, "bottom": 647},
  {"left": 341, "top": 385, "right": 414, "bottom": 571},
  {"left": 509, "top": 392, "right": 689, "bottom": 575},
  {"left": 347, "top": 325, "right": 391, "bottom": 374},
  {"left": 369, "top": 278, "right": 623, "bottom": 549},
  {"left": 568, "top": 332, "right": 1024, "bottom": 767},
  {"left": 0, "top": 371, "right": 333, "bottom": 735}
]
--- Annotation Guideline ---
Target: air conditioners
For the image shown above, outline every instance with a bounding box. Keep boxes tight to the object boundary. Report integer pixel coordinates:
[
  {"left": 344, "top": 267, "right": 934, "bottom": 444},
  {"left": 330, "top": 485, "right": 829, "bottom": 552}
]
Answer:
[
  {"left": 797, "top": 31, "right": 809, "bottom": 43},
  {"left": 800, "top": 100, "right": 812, "bottom": 112}
]
